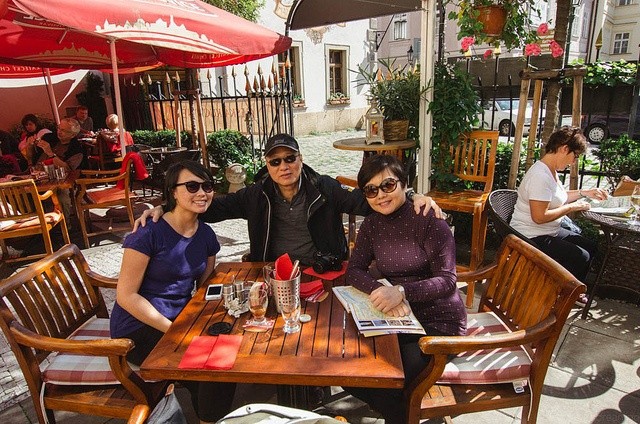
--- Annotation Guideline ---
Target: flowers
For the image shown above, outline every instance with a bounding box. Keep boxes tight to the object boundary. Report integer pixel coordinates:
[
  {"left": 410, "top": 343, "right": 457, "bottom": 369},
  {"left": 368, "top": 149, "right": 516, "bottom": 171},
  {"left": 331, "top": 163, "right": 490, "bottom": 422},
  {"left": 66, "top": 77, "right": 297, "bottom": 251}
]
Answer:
[
  {"left": 460, "top": 36, "right": 494, "bottom": 58},
  {"left": 526, "top": 22, "right": 563, "bottom": 57}
]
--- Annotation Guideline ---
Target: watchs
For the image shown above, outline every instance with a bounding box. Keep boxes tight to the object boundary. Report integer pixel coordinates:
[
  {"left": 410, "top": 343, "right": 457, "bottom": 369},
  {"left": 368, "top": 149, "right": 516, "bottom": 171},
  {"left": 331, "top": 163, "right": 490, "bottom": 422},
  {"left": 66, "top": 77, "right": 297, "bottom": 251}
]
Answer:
[
  {"left": 394, "top": 284, "right": 408, "bottom": 299},
  {"left": 409, "top": 191, "right": 423, "bottom": 200},
  {"left": 47, "top": 153, "right": 58, "bottom": 159}
]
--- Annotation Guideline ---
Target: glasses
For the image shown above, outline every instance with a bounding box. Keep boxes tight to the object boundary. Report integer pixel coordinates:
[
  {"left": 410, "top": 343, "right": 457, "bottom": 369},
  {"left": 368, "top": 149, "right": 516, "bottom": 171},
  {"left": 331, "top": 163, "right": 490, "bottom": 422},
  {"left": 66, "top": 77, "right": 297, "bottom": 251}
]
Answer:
[
  {"left": 175, "top": 180, "right": 214, "bottom": 192},
  {"left": 572, "top": 150, "right": 580, "bottom": 160},
  {"left": 57, "top": 124, "right": 72, "bottom": 132},
  {"left": 362, "top": 177, "right": 400, "bottom": 197},
  {"left": 266, "top": 154, "right": 299, "bottom": 165}
]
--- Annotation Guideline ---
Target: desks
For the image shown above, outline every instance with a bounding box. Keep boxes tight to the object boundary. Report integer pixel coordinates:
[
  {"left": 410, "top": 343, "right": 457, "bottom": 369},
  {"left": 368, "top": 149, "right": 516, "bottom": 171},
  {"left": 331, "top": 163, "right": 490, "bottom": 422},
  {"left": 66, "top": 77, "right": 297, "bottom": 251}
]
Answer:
[{"left": 334, "top": 138, "right": 416, "bottom": 165}]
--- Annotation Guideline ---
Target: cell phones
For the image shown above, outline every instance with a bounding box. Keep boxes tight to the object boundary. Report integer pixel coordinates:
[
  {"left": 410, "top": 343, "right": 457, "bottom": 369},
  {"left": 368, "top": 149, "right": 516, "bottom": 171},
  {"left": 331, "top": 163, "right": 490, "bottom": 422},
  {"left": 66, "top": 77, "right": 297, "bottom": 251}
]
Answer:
[{"left": 205, "top": 283, "right": 224, "bottom": 301}]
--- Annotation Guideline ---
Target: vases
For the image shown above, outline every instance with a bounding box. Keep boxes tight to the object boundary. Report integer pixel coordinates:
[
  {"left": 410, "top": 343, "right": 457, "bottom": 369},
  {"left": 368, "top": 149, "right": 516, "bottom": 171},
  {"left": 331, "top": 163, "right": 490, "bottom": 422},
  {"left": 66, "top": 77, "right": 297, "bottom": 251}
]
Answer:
[
  {"left": 470, "top": 56, "right": 497, "bottom": 86},
  {"left": 530, "top": 54, "right": 562, "bottom": 68}
]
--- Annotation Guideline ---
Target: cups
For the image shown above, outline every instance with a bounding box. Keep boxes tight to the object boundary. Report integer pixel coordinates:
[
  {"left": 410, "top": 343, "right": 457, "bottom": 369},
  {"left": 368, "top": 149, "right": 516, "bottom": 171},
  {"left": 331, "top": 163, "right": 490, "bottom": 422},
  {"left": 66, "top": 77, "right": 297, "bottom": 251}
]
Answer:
[
  {"left": 247, "top": 290, "right": 268, "bottom": 326},
  {"left": 280, "top": 295, "right": 301, "bottom": 334}
]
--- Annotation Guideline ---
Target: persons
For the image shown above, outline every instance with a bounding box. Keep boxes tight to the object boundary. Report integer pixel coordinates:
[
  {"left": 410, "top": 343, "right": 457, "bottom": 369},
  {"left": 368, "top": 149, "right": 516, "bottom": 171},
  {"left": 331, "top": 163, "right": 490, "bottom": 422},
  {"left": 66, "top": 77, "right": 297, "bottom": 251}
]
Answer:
[
  {"left": 131, "top": 134, "right": 447, "bottom": 265},
  {"left": 109, "top": 160, "right": 238, "bottom": 424},
  {"left": 1, "top": 130, "right": 28, "bottom": 178},
  {"left": 101, "top": 114, "right": 134, "bottom": 155},
  {"left": 509, "top": 126, "right": 607, "bottom": 308},
  {"left": 27, "top": 119, "right": 92, "bottom": 240},
  {"left": 69, "top": 106, "right": 94, "bottom": 132},
  {"left": 19, "top": 114, "right": 41, "bottom": 149},
  {"left": 340, "top": 154, "right": 466, "bottom": 424}
]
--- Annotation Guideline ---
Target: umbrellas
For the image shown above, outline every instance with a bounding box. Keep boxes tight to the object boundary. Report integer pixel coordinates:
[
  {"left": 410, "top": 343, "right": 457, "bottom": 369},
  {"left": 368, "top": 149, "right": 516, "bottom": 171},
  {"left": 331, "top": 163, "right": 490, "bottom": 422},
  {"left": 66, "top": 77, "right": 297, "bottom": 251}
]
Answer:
[
  {"left": 1, "top": 0, "right": 292, "bottom": 156},
  {"left": 1, "top": 61, "right": 162, "bottom": 125}
]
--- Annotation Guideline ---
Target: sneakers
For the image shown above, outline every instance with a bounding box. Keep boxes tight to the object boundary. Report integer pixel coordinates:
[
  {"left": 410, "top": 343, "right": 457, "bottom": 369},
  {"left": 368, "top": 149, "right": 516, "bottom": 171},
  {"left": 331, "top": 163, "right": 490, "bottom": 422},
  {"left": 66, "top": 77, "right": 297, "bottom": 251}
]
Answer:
[{"left": 575, "top": 292, "right": 598, "bottom": 308}]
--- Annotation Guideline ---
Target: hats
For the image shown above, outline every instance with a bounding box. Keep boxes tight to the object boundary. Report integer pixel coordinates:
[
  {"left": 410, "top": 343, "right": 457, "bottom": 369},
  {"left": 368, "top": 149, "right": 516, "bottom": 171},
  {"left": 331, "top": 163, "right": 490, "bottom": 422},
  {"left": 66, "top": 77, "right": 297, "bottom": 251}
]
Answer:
[{"left": 264, "top": 134, "right": 299, "bottom": 155}]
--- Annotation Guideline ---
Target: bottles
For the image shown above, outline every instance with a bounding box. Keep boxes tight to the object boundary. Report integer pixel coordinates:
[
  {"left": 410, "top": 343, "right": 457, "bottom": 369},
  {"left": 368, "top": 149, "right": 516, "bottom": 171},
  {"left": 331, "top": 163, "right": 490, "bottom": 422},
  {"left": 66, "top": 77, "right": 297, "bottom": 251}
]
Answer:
[
  {"left": 234, "top": 281, "right": 245, "bottom": 303},
  {"left": 54, "top": 167, "right": 61, "bottom": 180},
  {"left": 221, "top": 284, "right": 234, "bottom": 310},
  {"left": 59, "top": 166, "right": 66, "bottom": 177}
]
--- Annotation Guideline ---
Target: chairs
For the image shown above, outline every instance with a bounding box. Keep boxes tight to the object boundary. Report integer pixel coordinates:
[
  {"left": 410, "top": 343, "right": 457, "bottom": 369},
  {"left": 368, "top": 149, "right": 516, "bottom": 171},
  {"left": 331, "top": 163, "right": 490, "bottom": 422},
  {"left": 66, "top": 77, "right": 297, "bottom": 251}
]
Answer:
[
  {"left": 422, "top": 129, "right": 500, "bottom": 307},
  {"left": 487, "top": 188, "right": 540, "bottom": 248},
  {"left": 0, "top": 178, "right": 73, "bottom": 270},
  {"left": 74, "top": 157, "right": 136, "bottom": 248},
  {"left": 402, "top": 233, "right": 588, "bottom": 423},
  {"left": 1, "top": 244, "right": 168, "bottom": 424},
  {"left": 335, "top": 175, "right": 359, "bottom": 255}
]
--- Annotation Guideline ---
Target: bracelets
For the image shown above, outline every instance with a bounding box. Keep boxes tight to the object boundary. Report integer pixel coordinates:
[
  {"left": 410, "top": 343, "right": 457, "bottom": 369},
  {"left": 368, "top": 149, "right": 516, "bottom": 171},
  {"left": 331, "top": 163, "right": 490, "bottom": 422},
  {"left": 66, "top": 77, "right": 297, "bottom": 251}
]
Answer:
[{"left": 575, "top": 188, "right": 586, "bottom": 198}]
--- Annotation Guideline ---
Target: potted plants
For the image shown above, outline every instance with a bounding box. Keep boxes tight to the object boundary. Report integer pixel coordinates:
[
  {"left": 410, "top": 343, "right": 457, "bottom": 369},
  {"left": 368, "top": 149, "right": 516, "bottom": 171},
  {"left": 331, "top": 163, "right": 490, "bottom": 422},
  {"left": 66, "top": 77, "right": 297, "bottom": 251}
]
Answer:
[
  {"left": 349, "top": 56, "right": 432, "bottom": 140},
  {"left": 449, "top": 0, "right": 529, "bottom": 46}
]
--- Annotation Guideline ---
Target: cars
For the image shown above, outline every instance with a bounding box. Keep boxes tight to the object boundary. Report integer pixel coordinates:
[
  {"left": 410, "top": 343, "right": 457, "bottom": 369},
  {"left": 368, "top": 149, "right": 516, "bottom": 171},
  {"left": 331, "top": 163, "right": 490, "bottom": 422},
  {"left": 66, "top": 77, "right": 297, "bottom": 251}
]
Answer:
[
  {"left": 474, "top": 96, "right": 546, "bottom": 136},
  {"left": 581, "top": 111, "right": 640, "bottom": 145}
]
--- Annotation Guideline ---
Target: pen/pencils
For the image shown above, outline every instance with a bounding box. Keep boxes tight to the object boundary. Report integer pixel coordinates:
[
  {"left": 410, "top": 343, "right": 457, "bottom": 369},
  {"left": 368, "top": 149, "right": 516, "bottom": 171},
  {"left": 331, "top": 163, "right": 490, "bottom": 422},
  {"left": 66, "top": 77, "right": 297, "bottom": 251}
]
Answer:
[
  {"left": 343, "top": 309, "right": 346, "bottom": 328},
  {"left": 314, "top": 290, "right": 324, "bottom": 300}
]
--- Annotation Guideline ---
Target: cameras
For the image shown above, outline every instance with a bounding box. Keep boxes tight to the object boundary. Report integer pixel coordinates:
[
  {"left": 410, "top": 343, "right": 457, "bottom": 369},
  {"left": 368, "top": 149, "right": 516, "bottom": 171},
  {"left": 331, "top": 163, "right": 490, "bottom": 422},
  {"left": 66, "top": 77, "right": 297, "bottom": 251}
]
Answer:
[{"left": 311, "top": 250, "right": 345, "bottom": 274}]
